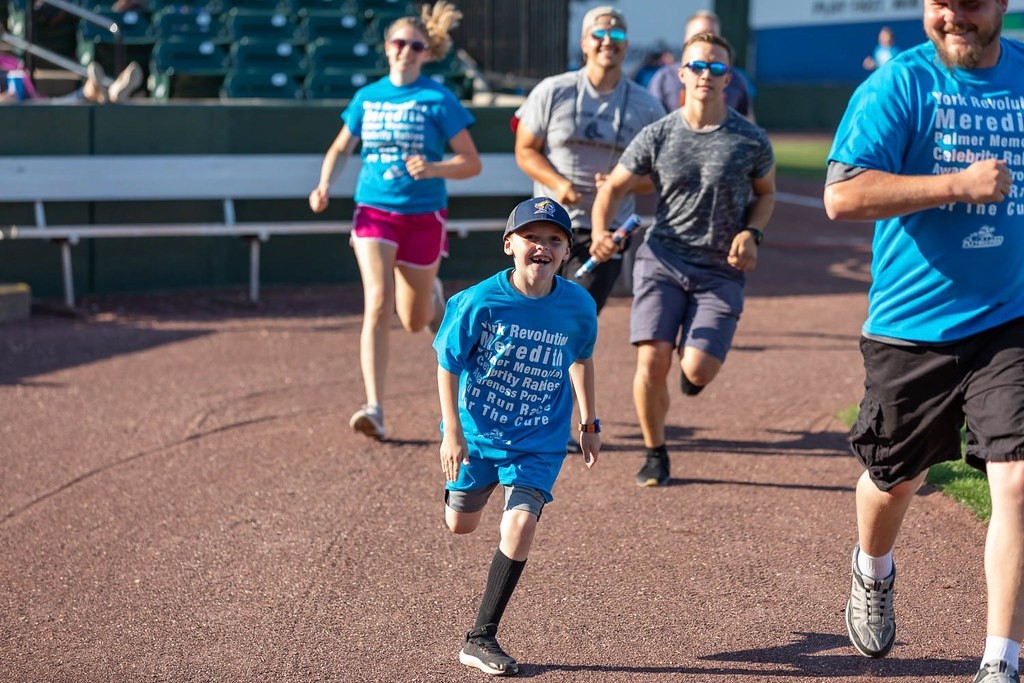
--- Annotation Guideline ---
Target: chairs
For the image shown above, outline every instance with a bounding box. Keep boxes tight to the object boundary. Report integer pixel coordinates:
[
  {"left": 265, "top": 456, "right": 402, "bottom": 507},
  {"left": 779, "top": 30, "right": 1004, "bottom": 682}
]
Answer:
[{"left": 0, "top": 0, "right": 481, "bottom": 103}]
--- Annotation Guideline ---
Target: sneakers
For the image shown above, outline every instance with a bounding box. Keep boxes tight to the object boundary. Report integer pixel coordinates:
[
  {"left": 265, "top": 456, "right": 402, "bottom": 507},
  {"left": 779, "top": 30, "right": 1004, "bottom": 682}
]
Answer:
[
  {"left": 972, "top": 660, "right": 1019, "bottom": 683},
  {"left": 350, "top": 402, "right": 385, "bottom": 438},
  {"left": 679, "top": 371, "right": 705, "bottom": 397},
  {"left": 636, "top": 451, "right": 671, "bottom": 486},
  {"left": 425, "top": 275, "right": 445, "bottom": 337},
  {"left": 459, "top": 623, "right": 518, "bottom": 676},
  {"left": 844, "top": 544, "right": 897, "bottom": 658}
]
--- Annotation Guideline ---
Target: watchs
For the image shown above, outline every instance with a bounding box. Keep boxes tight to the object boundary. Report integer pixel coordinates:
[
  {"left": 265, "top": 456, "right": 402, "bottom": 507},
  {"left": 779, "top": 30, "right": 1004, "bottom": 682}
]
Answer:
[
  {"left": 744, "top": 227, "right": 763, "bottom": 245},
  {"left": 578, "top": 418, "right": 603, "bottom": 433}
]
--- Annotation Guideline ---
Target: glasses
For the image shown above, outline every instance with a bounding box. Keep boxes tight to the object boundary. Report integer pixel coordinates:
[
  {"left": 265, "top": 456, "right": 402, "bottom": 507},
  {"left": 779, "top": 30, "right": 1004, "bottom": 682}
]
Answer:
[
  {"left": 589, "top": 28, "right": 628, "bottom": 43},
  {"left": 682, "top": 60, "right": 730, "bottom": 77},
  {"left": 388, "top": 38, "right": 430, "bottom": 52}
]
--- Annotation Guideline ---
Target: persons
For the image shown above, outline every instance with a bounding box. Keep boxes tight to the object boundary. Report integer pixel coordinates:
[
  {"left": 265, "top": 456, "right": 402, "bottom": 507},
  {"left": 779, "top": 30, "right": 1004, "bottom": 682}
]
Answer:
[
  {"left": 649, "top": 10, "right": 756, "bottom": 122},
  {"left": 0, "top": 0, "right": 140, "bottom": 105},
  {"left": 515, "top": 7, "right": 667, "bottom": 452},
  {"left": 308, "top": 17, "right": 482, "bottom": 442},
  {"left": 434, "top": 196, "right": 600, "bottom": 675},
  {"left": 419, "top": 0, "right": 484, "bottom": 100},
  {"left": 589, "top": 34, "right": 776, "bottom": 486},
  {"left": 823, "top": 0, "right": 1024, "bottom": 682}
]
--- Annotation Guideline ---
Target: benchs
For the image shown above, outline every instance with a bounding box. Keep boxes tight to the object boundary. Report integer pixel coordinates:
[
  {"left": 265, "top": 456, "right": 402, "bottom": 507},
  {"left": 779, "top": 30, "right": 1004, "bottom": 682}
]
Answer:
[{"left": 1, "top": 156, "right": 537, "bottom": 308}]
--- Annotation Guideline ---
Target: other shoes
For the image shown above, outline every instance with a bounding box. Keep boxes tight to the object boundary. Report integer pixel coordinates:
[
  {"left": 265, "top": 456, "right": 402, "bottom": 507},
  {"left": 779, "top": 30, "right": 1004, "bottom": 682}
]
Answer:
[
  {"left": 105, "top": 61, "right": 142, "bottom": 103},
  {"left": 85, "top": 58, "right": 109, "bottom": 105}
]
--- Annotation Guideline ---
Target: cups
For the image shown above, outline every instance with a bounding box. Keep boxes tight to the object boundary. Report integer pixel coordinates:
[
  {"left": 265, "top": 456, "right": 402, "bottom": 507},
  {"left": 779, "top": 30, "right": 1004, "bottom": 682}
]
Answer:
[{"left": 6, "top": 69, "right": 27, "bottom": 100}]
